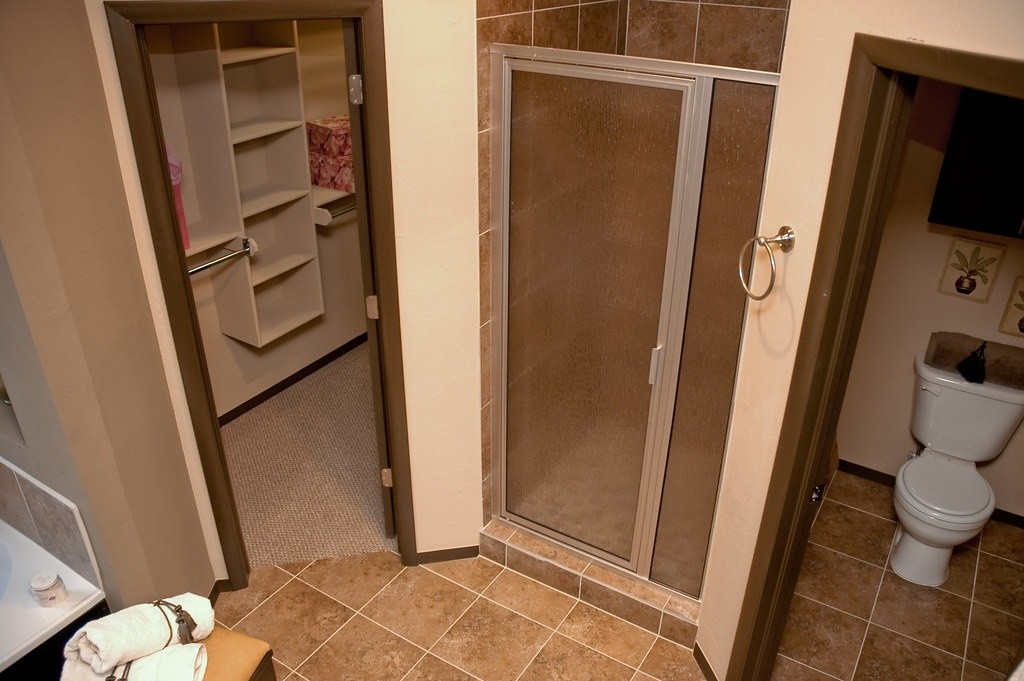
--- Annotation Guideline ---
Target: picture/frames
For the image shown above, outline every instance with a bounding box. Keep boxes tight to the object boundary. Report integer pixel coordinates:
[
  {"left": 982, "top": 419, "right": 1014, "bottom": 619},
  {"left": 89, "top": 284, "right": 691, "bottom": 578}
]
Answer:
[
  {"left": 936, "top": 239, "right": 1008, "bottom": 306},
  {"left": 998, "top": 275, "right": 1024, "bottom": 339}
]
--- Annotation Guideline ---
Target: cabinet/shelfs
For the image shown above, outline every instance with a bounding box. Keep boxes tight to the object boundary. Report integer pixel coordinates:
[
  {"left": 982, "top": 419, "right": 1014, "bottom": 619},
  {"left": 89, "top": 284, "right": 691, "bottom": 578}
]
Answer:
[{"left": 169, "top": 17, "right": 362, "bottom": 350}]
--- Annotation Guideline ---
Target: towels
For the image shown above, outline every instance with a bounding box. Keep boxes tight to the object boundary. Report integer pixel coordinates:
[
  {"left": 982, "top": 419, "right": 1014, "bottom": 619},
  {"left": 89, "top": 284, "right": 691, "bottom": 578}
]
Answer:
[
  {"left": 58, "top": 591, "right": 216, "bottom": 681},
  {"left": 925, "top": 83, "right": 1023, "bottom": 243}
]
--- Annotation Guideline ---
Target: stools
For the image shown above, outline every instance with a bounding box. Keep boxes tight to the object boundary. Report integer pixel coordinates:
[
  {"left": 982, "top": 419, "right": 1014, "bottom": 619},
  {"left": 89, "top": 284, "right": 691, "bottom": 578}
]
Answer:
[{"left": 202, "top": 629, "right": 277, "bottom": 681}]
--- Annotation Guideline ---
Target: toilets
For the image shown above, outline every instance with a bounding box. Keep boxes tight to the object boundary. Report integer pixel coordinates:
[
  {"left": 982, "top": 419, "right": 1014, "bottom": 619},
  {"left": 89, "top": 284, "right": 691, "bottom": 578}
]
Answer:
[{"left": 889, "top": 348, "right": 1024, "bottom": 589}]
